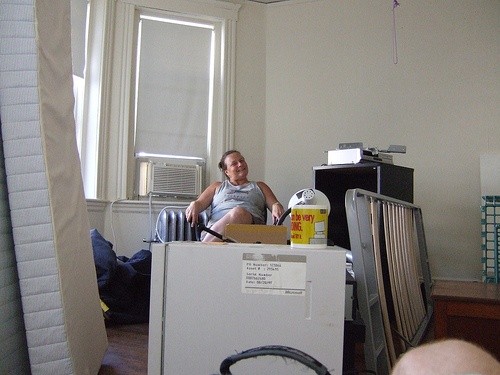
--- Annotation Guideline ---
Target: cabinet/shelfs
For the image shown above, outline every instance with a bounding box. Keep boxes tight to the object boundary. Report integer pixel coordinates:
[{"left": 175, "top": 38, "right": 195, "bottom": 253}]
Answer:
[{"left": 312, "top": 161, "right": 414, "bottom": 251}]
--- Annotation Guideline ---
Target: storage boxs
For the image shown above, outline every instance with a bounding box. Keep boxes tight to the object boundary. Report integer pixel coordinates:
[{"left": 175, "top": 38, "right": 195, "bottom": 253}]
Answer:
[{"left": 225, "top": 224, "right": 288, "bottom": 245}]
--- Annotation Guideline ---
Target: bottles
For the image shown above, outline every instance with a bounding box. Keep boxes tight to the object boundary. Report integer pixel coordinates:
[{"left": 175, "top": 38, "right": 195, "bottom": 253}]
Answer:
[{"left": 290, "top": 204, "right": 328, "bottom": 249}]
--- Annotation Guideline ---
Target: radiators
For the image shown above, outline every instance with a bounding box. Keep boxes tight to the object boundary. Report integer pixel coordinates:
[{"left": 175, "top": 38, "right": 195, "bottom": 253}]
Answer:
[{"left": 159, "top": 210, "right": 208, "bottom": 242}]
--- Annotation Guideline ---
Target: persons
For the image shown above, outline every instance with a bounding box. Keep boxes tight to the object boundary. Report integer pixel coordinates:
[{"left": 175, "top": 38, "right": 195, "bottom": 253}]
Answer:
[
  {"left": 184, "top": 150, "right": 285, "bottom": 243},
  {"left": 389, "top": 339, "right": 500, "bottom": 375}
]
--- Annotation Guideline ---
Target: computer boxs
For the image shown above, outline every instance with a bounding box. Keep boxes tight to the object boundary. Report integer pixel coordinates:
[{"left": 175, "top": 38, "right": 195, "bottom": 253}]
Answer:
[{"left": 146, "top": 241, "right": 345, "bottom": 375}]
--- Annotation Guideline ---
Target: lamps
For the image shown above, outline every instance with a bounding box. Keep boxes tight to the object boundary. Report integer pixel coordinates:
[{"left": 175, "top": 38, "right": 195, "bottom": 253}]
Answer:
[{"left": 276, "top": 189, "right": 330, "bottom": 225}]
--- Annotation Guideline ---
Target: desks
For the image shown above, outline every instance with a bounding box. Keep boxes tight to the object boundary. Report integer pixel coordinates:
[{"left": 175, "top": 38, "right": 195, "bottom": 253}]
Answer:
[{"left": 430, "top": 281, "right": 500, "bottom": 361}]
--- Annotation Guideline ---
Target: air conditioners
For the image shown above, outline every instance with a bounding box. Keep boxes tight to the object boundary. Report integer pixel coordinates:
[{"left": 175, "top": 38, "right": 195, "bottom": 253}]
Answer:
[{"left": 134, "top": 155, "right": 206, "bottom": 199}]
